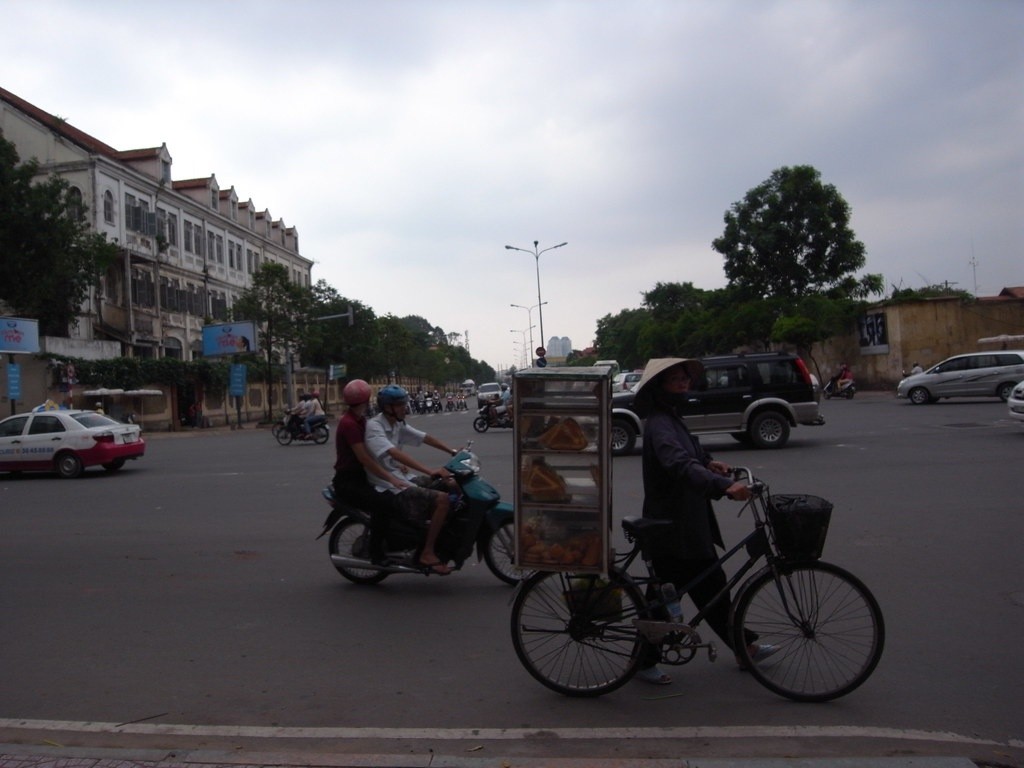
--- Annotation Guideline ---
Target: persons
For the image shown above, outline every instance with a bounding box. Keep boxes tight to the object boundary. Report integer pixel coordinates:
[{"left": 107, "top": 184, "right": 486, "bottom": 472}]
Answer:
[
  {"left": 406, "top": 388, "right": 469, "bottom": 413},
  {"left": 189, "top": 401, "right": 202, "bottom": 429},
  {"left": 235, "top": 336, "right": 250, "bottom": 351},
  {"left": 867, "top": 323, "right": 876, "bottom": 346},
  {"left": 626, "top": 358, "right": 781, "bottom": 685},
  {"left": 96, "top": 402, "right": 104, "bottom": 413},
  {"left": 831, "top": 364, "right": 853, "bottom": 392},
  {"left": 331, "top": 380, "right": 416, "bottom": 566},
  {"left": 284, "top": 391, "right": 325, "bottom": 438},
  {"left": 126, "top": 408, "right": 134, "bottom": 424},
  {"left": 364, "top": 385, "right": 465, "bottom": 576},
  {"left": 487, "top": 383, "right": 514, "bottom": 426},
  {"left": 903, "top": 361, "right": 922, "bottom": 376}
]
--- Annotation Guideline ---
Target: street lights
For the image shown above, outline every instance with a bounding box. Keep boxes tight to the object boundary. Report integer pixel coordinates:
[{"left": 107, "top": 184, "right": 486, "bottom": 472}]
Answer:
[
  {"left": 504, "top": 238, "right": 569, "bottom": 361},
  {"left": 510, "top": 301, "right": 548, "bottom": 369},
  {"left": 510, "top": 325, "right": 536, "bottom": 372}
]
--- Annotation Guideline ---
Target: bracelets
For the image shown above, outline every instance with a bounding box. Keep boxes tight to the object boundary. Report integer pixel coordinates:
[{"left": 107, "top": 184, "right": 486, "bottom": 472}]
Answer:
[{"left": 452, "top": 449, "right": 457, "bottom": 456}]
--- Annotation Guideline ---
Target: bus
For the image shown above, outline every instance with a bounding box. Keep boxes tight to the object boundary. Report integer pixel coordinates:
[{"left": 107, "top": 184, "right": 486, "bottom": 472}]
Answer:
[{"left": 459, "top": 379, "right": 476, "bottom": 396}]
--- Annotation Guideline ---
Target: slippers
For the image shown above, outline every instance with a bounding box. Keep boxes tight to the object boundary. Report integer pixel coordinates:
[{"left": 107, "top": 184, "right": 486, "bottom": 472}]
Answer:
[
  {"left": 737, "top": 644, "right": 780, "bottom": 671},
  {"left": 635, "top": 666, "right": 672, "bottom": 685},
  {"left": 420, "top": 559, "right": 451, "bottom": 576}
]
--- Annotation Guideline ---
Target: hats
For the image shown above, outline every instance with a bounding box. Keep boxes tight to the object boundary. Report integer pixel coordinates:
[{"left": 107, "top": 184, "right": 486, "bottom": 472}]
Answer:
[{"left": 631, "top": 356, "right": 703, "bottom": 408}]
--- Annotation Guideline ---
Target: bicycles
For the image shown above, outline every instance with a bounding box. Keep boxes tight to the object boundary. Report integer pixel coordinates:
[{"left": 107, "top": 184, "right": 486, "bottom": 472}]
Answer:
[{"left": 510, "top": 467, "right": 886, "bottom": 703}]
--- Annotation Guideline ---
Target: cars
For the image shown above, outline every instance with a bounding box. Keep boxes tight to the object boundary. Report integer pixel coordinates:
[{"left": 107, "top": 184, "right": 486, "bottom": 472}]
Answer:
[
  {"left": 0, "top": 399, "right": 145, "bottom": 480},
  {"left": 591, "top": 359, "right": 645, "bottom": 393},
  {"left": 1006, "top": 380, "right": 1024, "bottom": 422}
]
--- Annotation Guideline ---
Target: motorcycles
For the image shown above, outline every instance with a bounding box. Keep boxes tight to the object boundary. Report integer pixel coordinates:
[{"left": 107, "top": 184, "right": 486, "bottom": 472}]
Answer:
[
  {"left": 473, "top": 399, "right": 514, "bottom": 433},
  {"left": 315, "top": 440, "right": 549, "bottom": 587},
  {"left": 271, "top": 409, "right": 330, "bottom": 445}
]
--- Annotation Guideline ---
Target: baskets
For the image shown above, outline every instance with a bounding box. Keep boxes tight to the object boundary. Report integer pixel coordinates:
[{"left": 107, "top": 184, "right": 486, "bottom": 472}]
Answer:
[{"left": 765, "top": 494, "right": 833, "bottom": 559}]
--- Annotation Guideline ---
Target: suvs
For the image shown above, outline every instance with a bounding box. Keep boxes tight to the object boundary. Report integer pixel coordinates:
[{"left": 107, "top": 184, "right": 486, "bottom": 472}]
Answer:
[
  {"left": 612, "top": 350, "right": 826, "bottom": 457},
  {"left": 477, "top": 383, "right": 502, "bottom": 409}
]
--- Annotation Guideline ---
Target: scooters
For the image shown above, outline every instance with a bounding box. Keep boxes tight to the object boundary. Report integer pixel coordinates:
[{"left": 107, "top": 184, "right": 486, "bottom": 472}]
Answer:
[
  {"left": 823, "top": 375, "right": 857, "bottom": 399},
  {"left": 367, "top": 394, "right": 465, "bottom": 419}
]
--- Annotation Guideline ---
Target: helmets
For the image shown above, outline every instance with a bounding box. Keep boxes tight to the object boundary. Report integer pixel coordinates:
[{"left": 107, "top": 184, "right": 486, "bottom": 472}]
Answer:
[
  {"left": 501, "top": 383, "right": 508, "bottom": 389},
  {"left": 412, "top": 387, "right": 465, "bottom": 398},
  {"left": 299, "top": 393, "right": 304, "bottom": 401},
  {"left": 843, "top": 364, "right": 847, "bottom": 369},
  {"left": 376, "top": 385, "right": 409, "bottom": 411},
  {"left": 312, "top": 390, "right": 320, "bottom": 398},
  {"left": 839, "top": 362, "right": 843, "bottom": 368},
  {"left": 912, "top": 361, "right": 918, "bottom": 367},
  {"left": 303, "top": 392, "right": 312, "bottom": 401},
  {"left": 342, "top": 378, "right": 372, "bottom": 407}
]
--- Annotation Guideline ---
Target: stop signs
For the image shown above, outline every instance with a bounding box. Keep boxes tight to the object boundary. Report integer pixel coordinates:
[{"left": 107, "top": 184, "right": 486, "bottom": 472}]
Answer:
[{"left": 535, "top": 346, "right": 546, "bottom": 356}]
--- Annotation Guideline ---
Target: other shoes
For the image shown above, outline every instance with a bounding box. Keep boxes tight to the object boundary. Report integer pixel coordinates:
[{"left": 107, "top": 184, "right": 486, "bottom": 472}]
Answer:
[
  {"left": 296, "top": 434, "right": 305, "bottom": 439},
  {"left": 304, "top": 433, "right": 312, "bottom": 440},
  {"left": 372, "top": 557, "right": 391, "bottom": 567}
]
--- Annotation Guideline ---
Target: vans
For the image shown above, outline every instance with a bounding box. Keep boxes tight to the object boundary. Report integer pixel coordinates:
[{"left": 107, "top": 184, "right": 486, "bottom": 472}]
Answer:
[{"left": 895, "top": 350, "right": 1024, "bottom": 405}]
